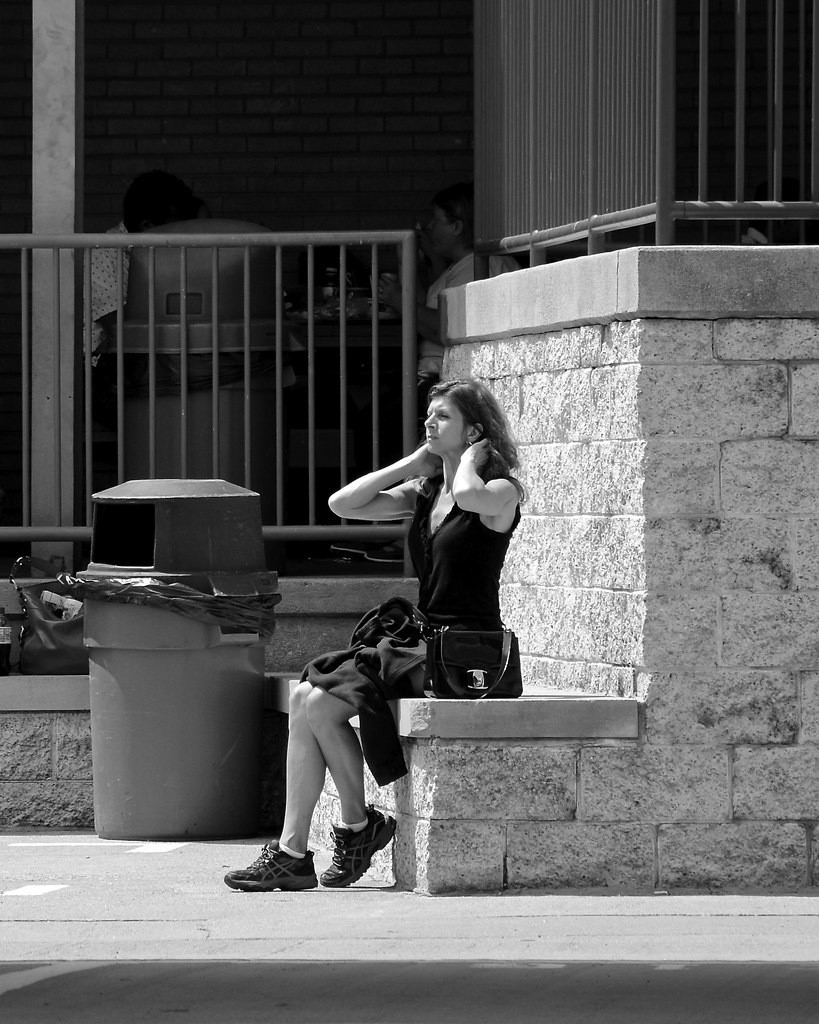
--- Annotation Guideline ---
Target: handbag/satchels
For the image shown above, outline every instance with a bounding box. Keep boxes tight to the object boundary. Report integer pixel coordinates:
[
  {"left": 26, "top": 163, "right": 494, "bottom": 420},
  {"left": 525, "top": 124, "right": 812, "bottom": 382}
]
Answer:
[{"left": 423, "top": 627, "right": 523, "bottom": 699}]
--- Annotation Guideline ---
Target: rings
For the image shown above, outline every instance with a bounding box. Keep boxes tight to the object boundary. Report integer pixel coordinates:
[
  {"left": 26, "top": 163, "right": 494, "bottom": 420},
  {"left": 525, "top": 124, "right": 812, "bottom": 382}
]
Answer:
[{"left": 379, "top": 289, "right": 383, "bottom": 293}]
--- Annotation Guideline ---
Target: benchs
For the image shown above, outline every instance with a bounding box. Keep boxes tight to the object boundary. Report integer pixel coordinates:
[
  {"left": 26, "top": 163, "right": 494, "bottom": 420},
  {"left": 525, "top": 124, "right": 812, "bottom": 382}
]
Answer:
[{"left": 261, "top": 670, "right": 641, "bottom": 900}]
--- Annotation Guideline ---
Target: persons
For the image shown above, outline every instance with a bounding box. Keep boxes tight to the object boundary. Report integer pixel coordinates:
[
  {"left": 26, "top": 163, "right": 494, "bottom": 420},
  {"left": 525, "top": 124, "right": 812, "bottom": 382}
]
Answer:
[
  {"left": 330, "top": 182, "right": 523, "bottom": 563},
  {"left": 83, "top": 169, "right": 213, "bottom": 436},
  {"left": 223, "top": 380, "right": 531, "bottom": 892},
  {"left": 741, "top": 176, "right": 809, "bottom": 245}
]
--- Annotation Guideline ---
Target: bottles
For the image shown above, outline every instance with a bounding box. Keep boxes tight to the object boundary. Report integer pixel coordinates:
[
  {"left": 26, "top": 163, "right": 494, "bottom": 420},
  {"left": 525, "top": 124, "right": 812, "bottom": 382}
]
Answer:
[{"left": 322, "top": 268, "right": 338, "bottom": 302}]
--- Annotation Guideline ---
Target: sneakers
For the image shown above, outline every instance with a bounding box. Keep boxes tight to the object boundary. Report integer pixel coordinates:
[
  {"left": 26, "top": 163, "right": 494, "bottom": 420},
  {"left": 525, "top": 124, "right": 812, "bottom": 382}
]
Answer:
[
  {"left": 320, "top": 804, "right": 398, "bottom": 887},
  {"left": 224, "top": 840, "right": 318, "bottom": 891}
]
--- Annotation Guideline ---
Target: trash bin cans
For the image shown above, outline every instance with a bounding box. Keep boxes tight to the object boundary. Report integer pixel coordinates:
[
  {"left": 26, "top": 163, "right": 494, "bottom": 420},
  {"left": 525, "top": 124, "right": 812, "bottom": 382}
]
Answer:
[{"left": 77, "top": 480, "right": 282, "bottom": 839}]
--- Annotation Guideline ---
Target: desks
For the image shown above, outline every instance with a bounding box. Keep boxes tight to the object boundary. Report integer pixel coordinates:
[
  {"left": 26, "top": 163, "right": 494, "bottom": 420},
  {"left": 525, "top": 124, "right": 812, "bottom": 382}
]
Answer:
[{"left": 70, "top": 318, "right": 402, "bottom": 531}]
